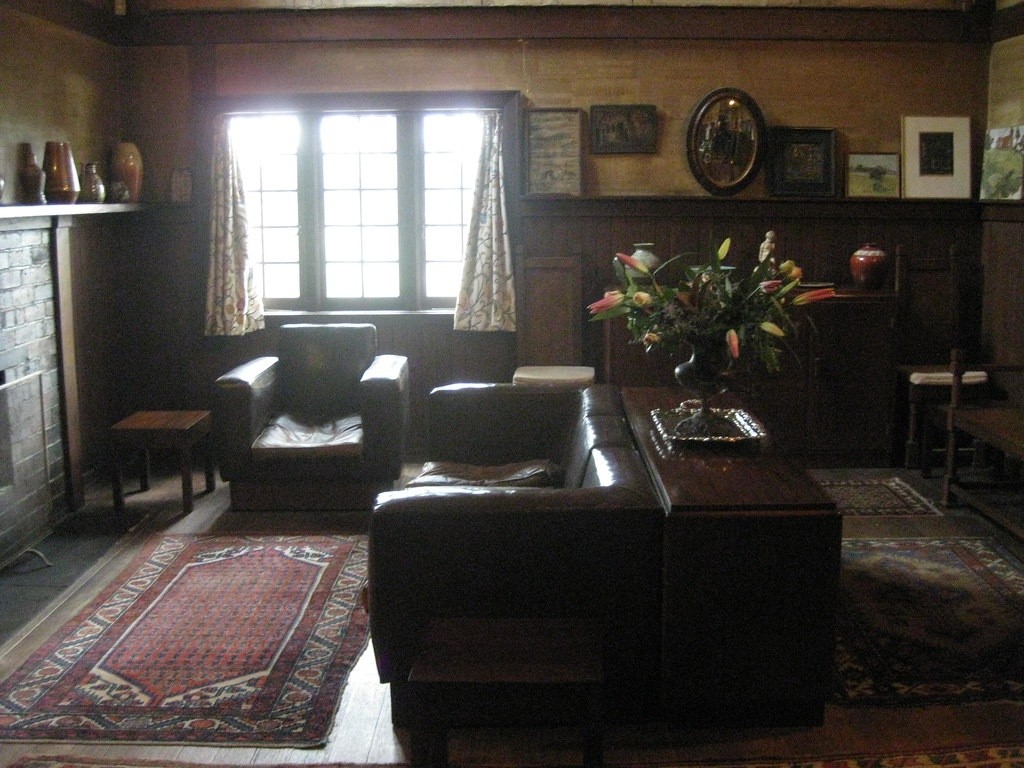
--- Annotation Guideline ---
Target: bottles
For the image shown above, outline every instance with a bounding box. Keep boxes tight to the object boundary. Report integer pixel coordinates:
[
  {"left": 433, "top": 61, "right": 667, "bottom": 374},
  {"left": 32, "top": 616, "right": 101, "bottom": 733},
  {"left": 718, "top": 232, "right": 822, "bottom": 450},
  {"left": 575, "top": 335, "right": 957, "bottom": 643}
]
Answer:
[
  {"left": 80, "top": 162, "right": 106, "bottom": 204},
  {"left": 20, "top": 151, "right": 48, "bottom": 206}
]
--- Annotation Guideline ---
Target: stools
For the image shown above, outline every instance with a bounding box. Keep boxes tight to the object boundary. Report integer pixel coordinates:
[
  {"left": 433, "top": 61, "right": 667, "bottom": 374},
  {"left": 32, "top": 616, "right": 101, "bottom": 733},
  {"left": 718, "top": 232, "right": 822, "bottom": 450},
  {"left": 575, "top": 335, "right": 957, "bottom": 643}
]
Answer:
[
  {"left": 406, "top": 614, "right": 605, "bottom": 768},
  {"left": 109, "top": 408, "right": 215, "bottom": 512}
]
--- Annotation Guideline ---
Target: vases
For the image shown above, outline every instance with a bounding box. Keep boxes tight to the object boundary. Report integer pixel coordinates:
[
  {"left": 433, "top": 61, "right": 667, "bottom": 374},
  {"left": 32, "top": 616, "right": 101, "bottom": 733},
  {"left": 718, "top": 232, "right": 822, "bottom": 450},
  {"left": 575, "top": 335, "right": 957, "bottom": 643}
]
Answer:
[
  {"left": 622, "top": 241, "right": 665, "bottom": 284},
  {"left": 14, "top": 150, "right": 50, "bottom": 202},
  {"left": 79, "top": 159, "right": 106, "bottom": 202},
  {"left": 109, "top": 140, "right": 145, "bottom": 202},
  {"left": 850, "top": 241, "right": 889, "bottom": 284},
  {"left": 672, "top": 333, "right": 730, "bottom": 391},
  {"left": 108, "top": 174, "right": 130, "bottom": 203}
]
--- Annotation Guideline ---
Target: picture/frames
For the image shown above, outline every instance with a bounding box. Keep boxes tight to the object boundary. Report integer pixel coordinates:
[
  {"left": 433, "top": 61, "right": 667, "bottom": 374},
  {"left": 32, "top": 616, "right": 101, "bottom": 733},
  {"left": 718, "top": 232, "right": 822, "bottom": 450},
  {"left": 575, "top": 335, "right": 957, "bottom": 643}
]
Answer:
[
  {"left": 43, "top": 139, "right": 81, "bottom": 202},
  {"left": 766, "top": 126, "right": 842, "bottom": 200},
  {"left": 520, "top": 107, "right": 587, "bottom": 201},
  {"left": 846, "top": 149, "right": 900, "bottom": 197},
  {"left": 590, "top": 101, "right": 660, "bottom": 154},
  {"left": 900, "top": 113, "right": 973, "bottom": 199}
]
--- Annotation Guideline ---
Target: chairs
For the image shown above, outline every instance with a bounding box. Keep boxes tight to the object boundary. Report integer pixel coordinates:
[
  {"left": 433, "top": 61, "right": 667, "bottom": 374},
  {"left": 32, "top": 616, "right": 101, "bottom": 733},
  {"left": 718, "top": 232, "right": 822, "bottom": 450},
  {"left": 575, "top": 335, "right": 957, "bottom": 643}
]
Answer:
[
  {"left": 937, "top": 348, "right": 1024, "bottom": 543},
  {"left": 893, "top": 241, "right": 1008, "bottom": 476}
]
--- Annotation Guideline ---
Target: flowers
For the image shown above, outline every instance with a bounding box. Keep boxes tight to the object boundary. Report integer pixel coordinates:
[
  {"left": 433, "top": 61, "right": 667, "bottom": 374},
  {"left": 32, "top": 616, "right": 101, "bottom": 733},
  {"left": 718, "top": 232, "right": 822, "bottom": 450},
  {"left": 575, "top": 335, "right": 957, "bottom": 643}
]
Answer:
[{"left": 576, "top": 235, "right": 837, "bottom": 373}]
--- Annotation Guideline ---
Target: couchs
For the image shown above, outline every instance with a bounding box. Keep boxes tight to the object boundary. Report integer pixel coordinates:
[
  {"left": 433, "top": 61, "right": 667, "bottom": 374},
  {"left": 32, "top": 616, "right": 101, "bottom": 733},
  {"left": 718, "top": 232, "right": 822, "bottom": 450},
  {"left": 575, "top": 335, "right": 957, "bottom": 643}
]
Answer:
[
  {"left": 351, "top": 361, "right": 662, "bottom": 736},
  {"left": 211, "top": 321, "right": 411, "bottom": 512}
]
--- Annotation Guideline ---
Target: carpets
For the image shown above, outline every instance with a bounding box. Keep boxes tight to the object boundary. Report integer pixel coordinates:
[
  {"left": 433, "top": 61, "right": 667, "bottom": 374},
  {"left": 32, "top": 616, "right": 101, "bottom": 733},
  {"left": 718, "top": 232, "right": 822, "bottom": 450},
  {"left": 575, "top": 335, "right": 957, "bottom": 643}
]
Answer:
[
  {"left": 0, "top": 525, "right": 373, "bottom": 750},
  {"left": 829, "top": 533, "right": 1024, "bottom": 709},
  {"left": 819, "top": 474, "right": 944, "bottom": 519}
]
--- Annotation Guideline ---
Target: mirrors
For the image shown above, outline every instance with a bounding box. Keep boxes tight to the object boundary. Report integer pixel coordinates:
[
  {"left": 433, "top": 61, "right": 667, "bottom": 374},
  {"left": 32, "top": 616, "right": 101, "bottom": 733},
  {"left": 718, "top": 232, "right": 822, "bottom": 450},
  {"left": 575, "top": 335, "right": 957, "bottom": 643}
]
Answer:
[{"left": 687, "top": 86, "right": 766, "bottom": 194}]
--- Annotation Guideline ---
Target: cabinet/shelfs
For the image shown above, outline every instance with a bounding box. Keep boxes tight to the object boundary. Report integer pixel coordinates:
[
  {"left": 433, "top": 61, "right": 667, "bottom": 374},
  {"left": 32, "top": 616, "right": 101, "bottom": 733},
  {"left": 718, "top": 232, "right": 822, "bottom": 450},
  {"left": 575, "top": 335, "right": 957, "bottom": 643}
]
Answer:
[
  {"left": 620, "top": 377, "right": 839, "bottom": 731},
  {"left": 599, "top": 281, "right": 903, "bottom": 470}
]
